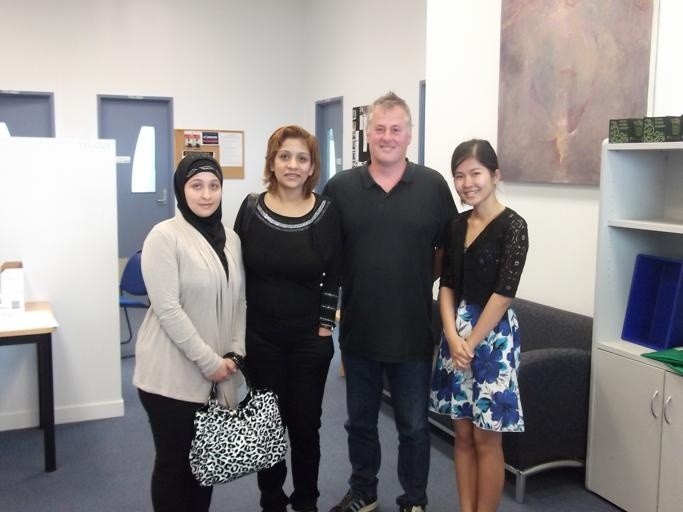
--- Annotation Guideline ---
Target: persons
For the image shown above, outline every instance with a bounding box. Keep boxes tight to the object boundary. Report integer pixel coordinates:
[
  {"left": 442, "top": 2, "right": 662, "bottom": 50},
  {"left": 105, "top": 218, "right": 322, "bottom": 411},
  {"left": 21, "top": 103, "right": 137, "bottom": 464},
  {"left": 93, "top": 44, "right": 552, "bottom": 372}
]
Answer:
[
  {"left": 131, "top": 154, "right": 249, "bottom": 512},
  {"left": 428, "top": 138, "right": 527, "bottom": 511},
  {"left": 233, "top": 123, "right": 338, "bottom": 512},
  {"left": 319, "top": 91, "right": 457, "bottom": 512}
]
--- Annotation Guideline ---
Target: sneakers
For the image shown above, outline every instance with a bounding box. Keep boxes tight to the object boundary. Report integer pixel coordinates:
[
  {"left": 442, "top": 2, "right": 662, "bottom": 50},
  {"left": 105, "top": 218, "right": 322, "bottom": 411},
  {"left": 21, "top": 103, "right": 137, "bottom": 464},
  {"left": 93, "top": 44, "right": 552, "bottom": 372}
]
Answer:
[{"left": 330, "top": 489, "right": 378, "bottom": 512}]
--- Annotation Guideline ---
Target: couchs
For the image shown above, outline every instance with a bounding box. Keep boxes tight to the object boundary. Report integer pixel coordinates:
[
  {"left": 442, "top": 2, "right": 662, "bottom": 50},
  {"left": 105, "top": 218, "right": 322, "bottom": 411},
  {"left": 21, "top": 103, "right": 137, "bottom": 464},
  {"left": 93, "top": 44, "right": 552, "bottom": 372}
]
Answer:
[{"left": 382, "top": 297, "right": 593, "bottom": 504}]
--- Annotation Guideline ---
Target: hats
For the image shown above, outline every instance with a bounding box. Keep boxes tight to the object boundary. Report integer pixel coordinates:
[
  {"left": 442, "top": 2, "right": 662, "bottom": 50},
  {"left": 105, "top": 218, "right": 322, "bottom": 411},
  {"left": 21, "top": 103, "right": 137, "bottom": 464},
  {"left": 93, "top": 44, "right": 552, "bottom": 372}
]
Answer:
[{"left": 183, "top": 159, "right": 222, "bottom": 184}]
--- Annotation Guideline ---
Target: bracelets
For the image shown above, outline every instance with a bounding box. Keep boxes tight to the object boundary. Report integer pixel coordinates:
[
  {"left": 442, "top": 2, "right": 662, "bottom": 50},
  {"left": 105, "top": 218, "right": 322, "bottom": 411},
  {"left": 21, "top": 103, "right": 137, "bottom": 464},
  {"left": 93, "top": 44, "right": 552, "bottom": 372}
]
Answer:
[
  {"left": 228, "top": 355, "right": 243, "bottom": 370},
  {"left": 318, "top": 323, "right": 334, "bottom": 332}
]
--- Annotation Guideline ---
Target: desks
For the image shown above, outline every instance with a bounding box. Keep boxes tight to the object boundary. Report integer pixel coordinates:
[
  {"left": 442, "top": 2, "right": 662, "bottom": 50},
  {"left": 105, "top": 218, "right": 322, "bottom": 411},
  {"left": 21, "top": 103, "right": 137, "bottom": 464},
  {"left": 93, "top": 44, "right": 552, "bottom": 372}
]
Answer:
[{"left": 0, "top": 302, "right": 60, "bottom": 472}]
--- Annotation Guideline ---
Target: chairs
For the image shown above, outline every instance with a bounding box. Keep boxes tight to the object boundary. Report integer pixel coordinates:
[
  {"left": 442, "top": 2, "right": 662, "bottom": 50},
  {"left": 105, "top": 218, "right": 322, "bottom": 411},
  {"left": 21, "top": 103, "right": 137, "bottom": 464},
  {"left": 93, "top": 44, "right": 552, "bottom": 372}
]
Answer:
[{"left": 121, "top": 249, "right": 151, "bottom": 359}]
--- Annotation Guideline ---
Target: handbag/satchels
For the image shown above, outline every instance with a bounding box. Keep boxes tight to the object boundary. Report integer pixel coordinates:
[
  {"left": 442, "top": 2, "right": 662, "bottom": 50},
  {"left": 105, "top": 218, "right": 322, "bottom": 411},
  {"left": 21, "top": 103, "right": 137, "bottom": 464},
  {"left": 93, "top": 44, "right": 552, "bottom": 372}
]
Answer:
[{"left": 189, "top": 390, "right": 287, "bottom": 488}]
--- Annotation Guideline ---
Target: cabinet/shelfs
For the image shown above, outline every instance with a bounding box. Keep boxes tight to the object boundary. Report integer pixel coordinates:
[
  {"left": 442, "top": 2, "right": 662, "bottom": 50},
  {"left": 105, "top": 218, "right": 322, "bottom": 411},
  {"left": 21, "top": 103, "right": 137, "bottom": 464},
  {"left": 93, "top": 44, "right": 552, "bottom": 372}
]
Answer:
[{"left": 585, "top": 138, "right": 683, "bottom": 512}]
